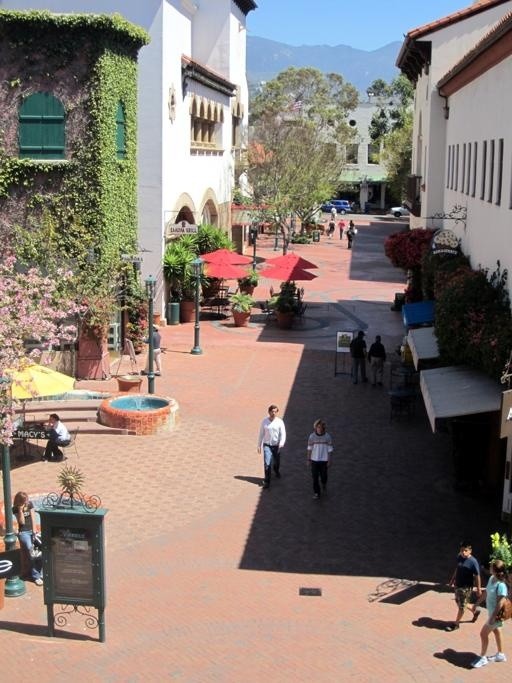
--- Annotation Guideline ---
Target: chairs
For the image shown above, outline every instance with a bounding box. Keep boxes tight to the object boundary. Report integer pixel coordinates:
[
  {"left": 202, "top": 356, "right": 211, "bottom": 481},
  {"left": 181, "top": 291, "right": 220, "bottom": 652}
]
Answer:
[
  {"left": 388, "top": 362, "right": 412, "bottom": 424},
  {"left": 258, "top": 280, "right": 308, "bottom": 325},
  {"left": 53, "top": 426, "right": 81, "bottom": 460}
]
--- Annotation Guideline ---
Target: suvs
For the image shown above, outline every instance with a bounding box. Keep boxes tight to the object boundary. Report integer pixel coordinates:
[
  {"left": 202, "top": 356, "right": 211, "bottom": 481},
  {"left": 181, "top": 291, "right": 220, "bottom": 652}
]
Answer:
[{"left": 322, "top": 200, "right": 350, "bottom": 215}]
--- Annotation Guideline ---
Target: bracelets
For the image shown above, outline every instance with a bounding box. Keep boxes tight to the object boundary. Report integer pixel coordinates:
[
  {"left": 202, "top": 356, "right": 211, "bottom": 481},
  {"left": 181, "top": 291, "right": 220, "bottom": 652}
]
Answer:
[{"left": 306, "top": 458, "right": 311, "bottom": 461}]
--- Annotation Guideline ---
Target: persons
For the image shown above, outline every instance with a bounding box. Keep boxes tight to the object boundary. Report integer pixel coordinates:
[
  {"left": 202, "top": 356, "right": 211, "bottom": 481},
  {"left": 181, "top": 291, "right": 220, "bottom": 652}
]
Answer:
[
  {"left": 470, "top": 560, "right": 508, "bottom": 668},
  {"left": 445, "top": 541, "right": 482, "bottom": 631},
  {"left": 327, "top": 208, "right": 354, "bottom": 248},
  {"left": 11, "top": 492, "right": 44, "bottom": 586},
  {"left": 307, "top": 418, "right": 334, "bottom": 499},
  {"left": 368, "top": 334, "right": 387, "bottom": 387},
  {"left": 140, "top": 324, "right": 161, "bottom": 376},
  {"left": 41, "top": 414, "right": 71, "bottom": 462},
  {"left": 257, "top": 405, "right": 286, "bottom": 489},
  {"left": 349, "top": 331, "right": 368, "bottom": 384}
]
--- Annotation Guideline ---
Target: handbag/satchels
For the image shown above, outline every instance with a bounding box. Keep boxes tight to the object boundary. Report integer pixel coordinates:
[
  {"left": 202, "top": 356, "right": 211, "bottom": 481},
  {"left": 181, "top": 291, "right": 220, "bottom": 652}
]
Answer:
[{"left": 497, "top": 597, "right": 512, "bottom": 621}]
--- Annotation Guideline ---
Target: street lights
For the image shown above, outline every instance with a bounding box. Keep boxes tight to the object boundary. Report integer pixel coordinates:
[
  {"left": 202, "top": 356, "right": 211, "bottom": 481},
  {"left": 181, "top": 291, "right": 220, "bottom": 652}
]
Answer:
[
  {"left": 143, "top": 275, "right": 158, "bottom": 394},
  {"left": 191, "top": 256, "right": 203, "bottom": 354},
  {"left": 249, "top": 228, "right": 257, "bottom": 271},
  {"left": 0, "top": 376, "right": 26, "bottom": 598}
]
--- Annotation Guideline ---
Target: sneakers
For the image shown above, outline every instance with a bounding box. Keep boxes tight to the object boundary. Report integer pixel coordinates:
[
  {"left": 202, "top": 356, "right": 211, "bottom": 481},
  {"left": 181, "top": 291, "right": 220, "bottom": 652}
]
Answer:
[
  {"left": 488, "top": 652, "right": 506, "bottom": 662},
  {"left": 470, "top": 656, "right": 488, "bottom": 667},
  {"left": 35, "top": 579, "right": 43, "bottom": 585},
  {"left": 472, "top": 610, "right": 480, "bottom": 623},
  {"left": 445, "top": 623, "right": 459, "bottom": 631}
]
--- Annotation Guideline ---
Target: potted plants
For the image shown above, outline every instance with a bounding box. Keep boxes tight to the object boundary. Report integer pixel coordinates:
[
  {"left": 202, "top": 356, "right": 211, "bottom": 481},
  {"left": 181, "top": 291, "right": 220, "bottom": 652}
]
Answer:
[
  {"left": 237, "top": 270, "right": 259, "bottom": 295},
  {"left": 202, "top": 229, "right": 237, "bottom": 299},
  {"left": 181, "top": 257, "right": 212, "bottom": 322},
  {"left": 231, "top": 292, "right": 255, "bottom": 327}
]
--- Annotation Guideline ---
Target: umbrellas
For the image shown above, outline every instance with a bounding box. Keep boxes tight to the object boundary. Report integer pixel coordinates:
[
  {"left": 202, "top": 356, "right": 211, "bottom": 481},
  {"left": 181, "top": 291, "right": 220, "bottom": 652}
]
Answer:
[
  {"left": 257, "top": 267, "right": 318, "bottom": 281},
  {"left": 199, "top": 249, "right": 253, "bottom": 265},
  {"left": 4, "top": 356, "right": 75, "bottom": 455},
  {"left": 266, "top": 253, "right": 319, "bottom": 284},
  {"left": 204, "top": 259, "right": 251, "bottom": 298}
]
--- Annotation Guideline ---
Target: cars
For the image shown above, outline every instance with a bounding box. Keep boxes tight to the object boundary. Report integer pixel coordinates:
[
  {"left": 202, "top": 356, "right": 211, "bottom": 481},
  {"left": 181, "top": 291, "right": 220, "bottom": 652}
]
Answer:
[{"left": 391, "top": 207, "right": 410, "bottom": 218}]
